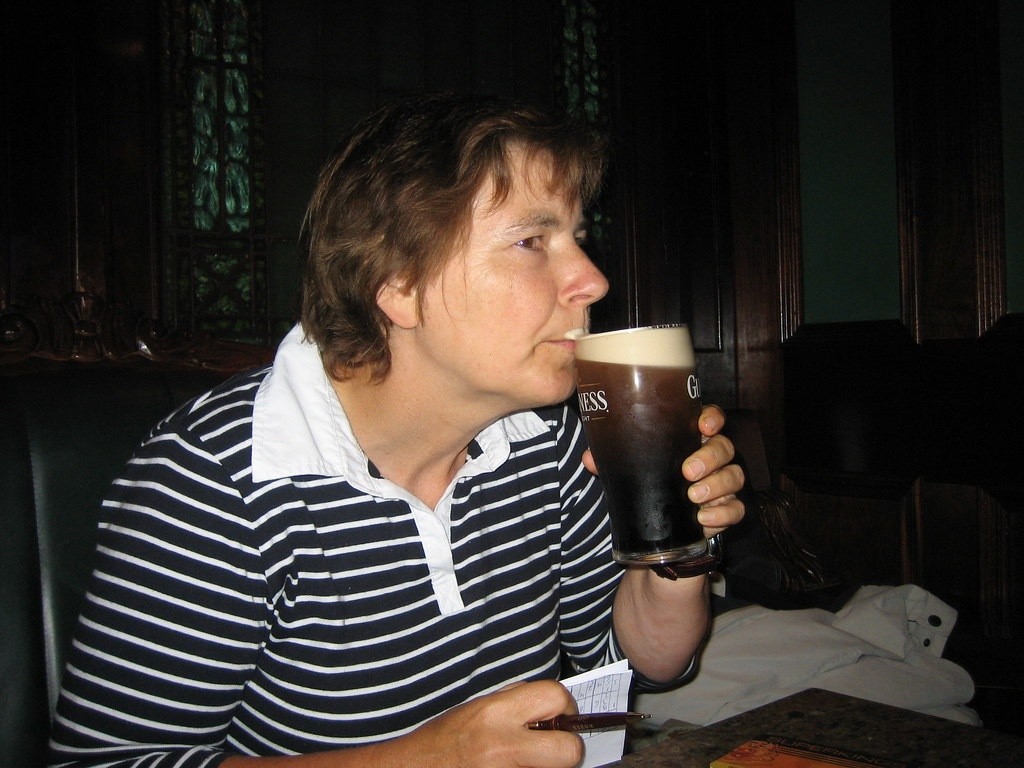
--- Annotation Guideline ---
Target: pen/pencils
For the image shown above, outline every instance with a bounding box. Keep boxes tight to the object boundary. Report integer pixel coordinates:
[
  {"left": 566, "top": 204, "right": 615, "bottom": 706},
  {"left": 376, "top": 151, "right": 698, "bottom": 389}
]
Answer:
[{"left": 529, "top": 711, "right": 653, "bottom": 732}]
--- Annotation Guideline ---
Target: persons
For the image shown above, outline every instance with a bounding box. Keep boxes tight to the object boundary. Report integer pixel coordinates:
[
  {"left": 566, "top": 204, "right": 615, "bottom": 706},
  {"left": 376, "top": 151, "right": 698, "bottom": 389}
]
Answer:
[{"left": 52, "top": 89, "right": 748, "bottom": 768}]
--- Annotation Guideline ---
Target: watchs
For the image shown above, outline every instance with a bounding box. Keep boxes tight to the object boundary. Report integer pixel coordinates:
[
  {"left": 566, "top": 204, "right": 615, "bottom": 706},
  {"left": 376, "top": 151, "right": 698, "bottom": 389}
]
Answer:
[{"left": 655, "top": 533, "right": 722, "bottom": 582}]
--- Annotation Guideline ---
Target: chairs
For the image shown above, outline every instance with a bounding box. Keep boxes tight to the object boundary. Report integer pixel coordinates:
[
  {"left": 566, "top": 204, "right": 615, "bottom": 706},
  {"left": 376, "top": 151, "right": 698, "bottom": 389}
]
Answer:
[
  {"left": 25, "top": 352, "right": 212, "bottom": 738},
  {"left": 781, "top": 306, "right": 1024, "bottom": 640}
]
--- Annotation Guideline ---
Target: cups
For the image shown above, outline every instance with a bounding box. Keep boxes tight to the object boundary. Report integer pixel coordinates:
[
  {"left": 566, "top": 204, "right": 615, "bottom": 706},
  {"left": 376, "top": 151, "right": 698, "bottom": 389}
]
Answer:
[{"left": 578, "top": 323, "right": 721, "bottom": 565}]
